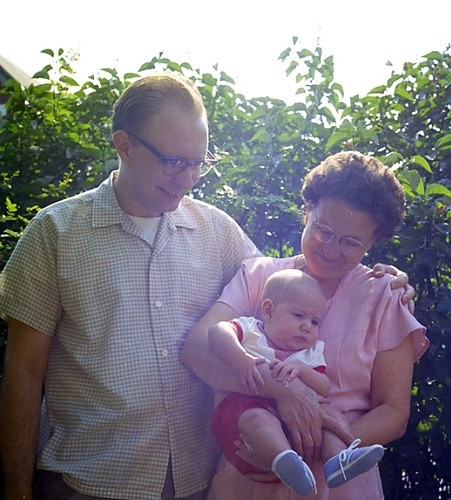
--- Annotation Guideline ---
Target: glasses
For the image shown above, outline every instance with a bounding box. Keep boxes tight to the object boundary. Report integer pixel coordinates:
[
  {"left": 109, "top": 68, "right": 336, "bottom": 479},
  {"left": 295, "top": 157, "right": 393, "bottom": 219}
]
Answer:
[
  {"left": 126, "top": 132, "right": 218, "bottom": 177},
  {"left": 311, "top": 205, "right": 379, "bottom": 257}
]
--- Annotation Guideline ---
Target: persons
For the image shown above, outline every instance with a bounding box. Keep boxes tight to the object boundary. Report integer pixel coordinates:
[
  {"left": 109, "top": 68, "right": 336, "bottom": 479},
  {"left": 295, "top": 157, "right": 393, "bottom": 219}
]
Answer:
[
  {"left": 180, "top": 146, "right": 427, "bottom": 498},
  {"left": 1, "top": 72, "right": 419, "bottom": 500},
  {"left": 208, "top": 271, "right": 384, "bottom": 497}
]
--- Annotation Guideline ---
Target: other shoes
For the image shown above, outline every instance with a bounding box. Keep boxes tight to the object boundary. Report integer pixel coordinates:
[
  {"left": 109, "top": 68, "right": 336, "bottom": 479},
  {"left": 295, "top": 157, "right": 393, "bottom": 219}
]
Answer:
[
  {"left": 322, "top": 438, "right": 384, "bottom": 488},
  {"left": 271, "top": 452, "right": 319, "bottom": 498}
]
